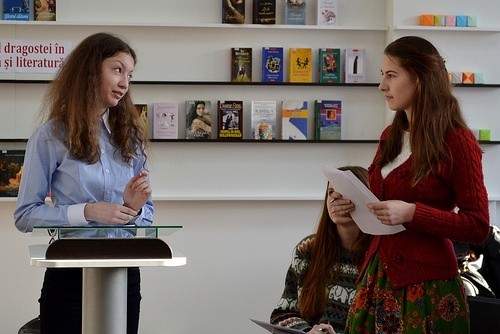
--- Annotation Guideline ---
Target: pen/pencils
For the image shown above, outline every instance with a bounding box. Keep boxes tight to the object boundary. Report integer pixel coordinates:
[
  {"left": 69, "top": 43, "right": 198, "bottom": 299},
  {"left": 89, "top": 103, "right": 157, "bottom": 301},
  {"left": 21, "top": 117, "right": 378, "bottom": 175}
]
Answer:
[{"left": 318, "top": 328, "right": 326, "bottom": 332}]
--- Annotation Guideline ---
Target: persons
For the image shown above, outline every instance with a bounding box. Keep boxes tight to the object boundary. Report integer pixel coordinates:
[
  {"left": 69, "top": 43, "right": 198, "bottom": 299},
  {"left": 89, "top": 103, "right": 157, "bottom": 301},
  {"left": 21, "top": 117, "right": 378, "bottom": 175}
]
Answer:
[
  {"left": 270, "top": 36, "right": 490, "bottom": 334},
  {"left": 13, "top": 32, "right": 154, "bottom": 333},
  {"left": 187, "top": 101, "right": 211, "bottom": 139},
  {"left": 224, "top": 111, "right": 233, "bottom": 129}
]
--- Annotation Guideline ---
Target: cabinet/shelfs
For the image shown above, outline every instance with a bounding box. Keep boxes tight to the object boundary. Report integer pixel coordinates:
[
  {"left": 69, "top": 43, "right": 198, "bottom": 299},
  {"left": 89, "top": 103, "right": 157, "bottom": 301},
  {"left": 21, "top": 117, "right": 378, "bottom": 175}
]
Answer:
[{"left": 1, "top": 0, "right": 500, "bottom": 204}]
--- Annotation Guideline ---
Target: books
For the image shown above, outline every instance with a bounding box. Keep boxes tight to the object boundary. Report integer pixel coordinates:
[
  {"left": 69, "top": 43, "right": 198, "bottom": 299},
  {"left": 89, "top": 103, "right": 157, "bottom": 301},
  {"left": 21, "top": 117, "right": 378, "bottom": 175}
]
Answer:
[
  {"left": 230, "top": 47, "right": 367, "bottom": 84},
  {"left": 134, "top": 100, "right": 342, "bottom": 141},
  {"left": 2, "top": 0, "right": 56, "bottom": 21},
  {"left": 222, "top": 0, "right": 340, "bottom": 25}
]
